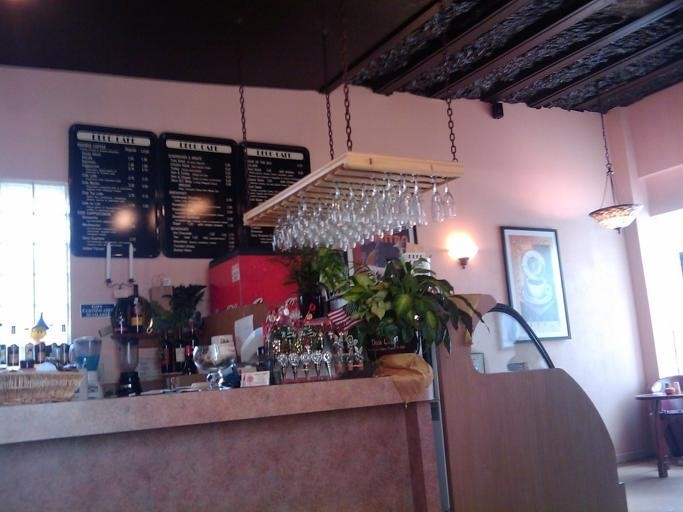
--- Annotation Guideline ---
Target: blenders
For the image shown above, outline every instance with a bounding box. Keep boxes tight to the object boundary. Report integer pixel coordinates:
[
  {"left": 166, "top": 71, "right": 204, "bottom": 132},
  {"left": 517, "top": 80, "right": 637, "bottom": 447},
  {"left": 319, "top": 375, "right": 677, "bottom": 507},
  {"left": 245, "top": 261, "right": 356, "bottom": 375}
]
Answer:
[
  {"left": 113, "top": 336, "right": 143, "bottom": 398},
  {"left": 69, "top": 335, "right": 105, "bottom": 399}
]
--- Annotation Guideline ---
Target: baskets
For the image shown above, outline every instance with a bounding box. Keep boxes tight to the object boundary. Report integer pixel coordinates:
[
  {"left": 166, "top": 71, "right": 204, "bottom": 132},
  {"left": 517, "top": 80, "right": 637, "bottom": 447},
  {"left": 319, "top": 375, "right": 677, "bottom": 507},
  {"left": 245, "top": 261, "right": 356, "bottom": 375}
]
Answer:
[{"left": 0, "top": 371, "right": 87, "bottom": 404}]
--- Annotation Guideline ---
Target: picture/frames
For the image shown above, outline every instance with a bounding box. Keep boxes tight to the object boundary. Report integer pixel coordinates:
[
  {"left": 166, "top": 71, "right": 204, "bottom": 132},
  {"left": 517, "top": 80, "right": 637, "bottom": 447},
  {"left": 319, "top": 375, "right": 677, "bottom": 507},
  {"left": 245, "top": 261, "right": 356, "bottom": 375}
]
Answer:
[{"left": 498, "top": 226, "right": 572, "bottom": 342}]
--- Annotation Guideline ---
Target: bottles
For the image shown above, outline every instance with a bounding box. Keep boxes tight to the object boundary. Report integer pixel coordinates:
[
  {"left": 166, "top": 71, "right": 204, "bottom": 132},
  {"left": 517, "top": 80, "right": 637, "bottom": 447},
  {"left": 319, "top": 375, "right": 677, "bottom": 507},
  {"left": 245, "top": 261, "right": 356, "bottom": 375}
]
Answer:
[
  {"left": 160, "top": 319, "right": 200, "bottom": 374},
  {"left": 111, "top": 284, "right": 144, "bottom": 334}
]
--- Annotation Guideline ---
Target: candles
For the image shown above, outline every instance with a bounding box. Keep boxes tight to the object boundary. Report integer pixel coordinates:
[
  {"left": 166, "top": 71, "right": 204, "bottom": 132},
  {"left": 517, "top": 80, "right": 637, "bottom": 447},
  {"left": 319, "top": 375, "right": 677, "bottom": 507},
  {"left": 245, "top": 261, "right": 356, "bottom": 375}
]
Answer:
[
  {"left": 129, "top": 245, "right": 134, "bottom": 279},
  {"left": 106, "top": 241, "right": 112, "bottom": 280}
]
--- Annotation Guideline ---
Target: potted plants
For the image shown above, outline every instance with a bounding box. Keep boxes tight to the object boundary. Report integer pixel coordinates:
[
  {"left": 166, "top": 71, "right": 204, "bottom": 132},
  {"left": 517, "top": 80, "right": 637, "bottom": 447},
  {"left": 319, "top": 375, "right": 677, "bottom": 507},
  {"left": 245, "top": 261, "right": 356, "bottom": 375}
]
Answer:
[
  {"left": 272, "top": 246, "right": 347, "bottom": 319},
  {"left": 346, "top": 259, "right": 489, "bottom": 361}
]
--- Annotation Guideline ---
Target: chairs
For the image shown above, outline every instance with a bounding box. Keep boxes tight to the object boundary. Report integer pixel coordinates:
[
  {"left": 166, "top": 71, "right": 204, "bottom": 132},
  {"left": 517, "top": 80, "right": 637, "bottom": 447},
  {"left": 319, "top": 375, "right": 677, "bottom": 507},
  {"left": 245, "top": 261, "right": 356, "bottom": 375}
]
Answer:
[{"left": 651, "top": 374, "right": 683, "bottom": 457}]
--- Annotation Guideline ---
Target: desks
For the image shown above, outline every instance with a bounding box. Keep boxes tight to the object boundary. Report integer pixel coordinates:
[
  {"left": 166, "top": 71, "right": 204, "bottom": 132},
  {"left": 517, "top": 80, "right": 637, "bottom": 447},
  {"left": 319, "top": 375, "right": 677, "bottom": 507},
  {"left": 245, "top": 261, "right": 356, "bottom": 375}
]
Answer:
[{"left": 637, "top": 393, "right": 683, "bottom": 479}]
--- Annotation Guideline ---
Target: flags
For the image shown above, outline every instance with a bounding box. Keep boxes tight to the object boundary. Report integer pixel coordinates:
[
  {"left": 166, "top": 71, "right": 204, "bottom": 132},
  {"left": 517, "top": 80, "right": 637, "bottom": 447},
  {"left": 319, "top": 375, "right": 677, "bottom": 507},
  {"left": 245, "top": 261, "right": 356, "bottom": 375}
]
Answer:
[{"left": 328, "top": 300, "right": 365, "bottom": 332}]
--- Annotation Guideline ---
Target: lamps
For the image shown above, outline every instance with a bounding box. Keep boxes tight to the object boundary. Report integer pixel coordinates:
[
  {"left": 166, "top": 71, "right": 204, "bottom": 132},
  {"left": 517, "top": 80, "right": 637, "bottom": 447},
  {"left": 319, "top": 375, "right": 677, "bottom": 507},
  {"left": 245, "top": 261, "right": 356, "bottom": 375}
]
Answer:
[
  {"left": 590, "top": 83, "right": 642, "bottom": 236},
  {"left": 447, "top": 231, "right": 476, "bottom": 268}
]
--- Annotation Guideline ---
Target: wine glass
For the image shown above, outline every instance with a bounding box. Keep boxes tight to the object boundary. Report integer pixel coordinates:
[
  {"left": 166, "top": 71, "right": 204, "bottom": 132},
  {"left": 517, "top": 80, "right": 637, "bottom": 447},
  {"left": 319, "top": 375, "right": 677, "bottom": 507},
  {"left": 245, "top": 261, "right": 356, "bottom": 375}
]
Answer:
[
  {"left": 272, "top": 173, "right": 457, "bottom": 252},
  {"left": 193, "top": 341, "right": 238, "bottom": 391}
]
